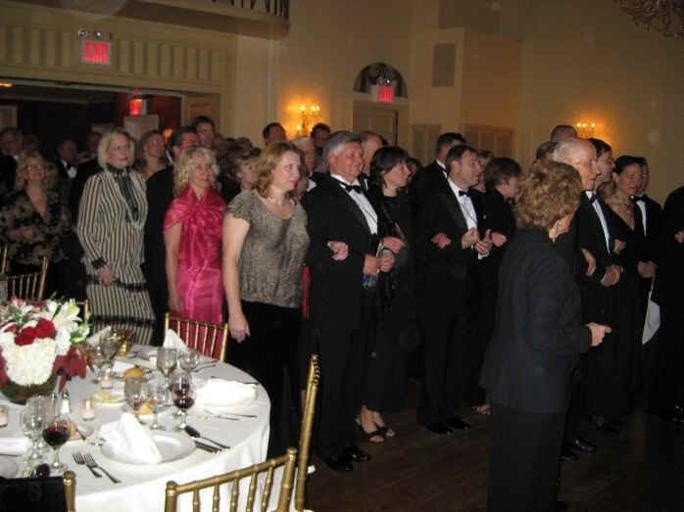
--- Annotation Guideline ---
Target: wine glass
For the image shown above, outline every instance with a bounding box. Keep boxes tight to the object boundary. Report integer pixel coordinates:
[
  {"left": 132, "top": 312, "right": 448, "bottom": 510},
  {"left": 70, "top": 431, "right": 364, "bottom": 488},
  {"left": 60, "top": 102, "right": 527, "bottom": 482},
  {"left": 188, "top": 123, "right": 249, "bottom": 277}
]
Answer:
[
  {"left": 143, "top": 380, "right": 171, "bottom": 433},
  {"left": 156, "top": 347, "right": 178, "bottom": 388},
  {"left": 124, "top": 376, "right": 149, "bottom": 425},
  {"left": 88, "top": 348, "right": 108, "bottom": 384},
  {"left": 19, "top": 408, "right": 47, "bottom": 462},
  {"left": 27, "top": 394, "right": 59, "bottom": 453},
  {"left": 171, "top": 387, "right": 196, "bottom": 431},
  {"left": 99, "top": 334, "right": 123, "bottom": 360},
  {"left": 42, "top": 407, "right": 69, "bottom": 472},
  {"left": 168, "top": 368, "right": 192, "bottom": 418},
  {"left": 179, "top": 348, "right": 199, "bottom": 377}
]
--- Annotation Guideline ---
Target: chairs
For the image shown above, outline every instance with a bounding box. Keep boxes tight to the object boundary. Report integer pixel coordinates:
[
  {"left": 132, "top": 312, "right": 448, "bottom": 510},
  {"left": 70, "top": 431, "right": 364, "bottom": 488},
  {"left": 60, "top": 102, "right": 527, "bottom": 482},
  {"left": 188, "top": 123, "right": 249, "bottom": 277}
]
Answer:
[
  {"left": 0, "top": 471, "right": 75, "bottom": 511},
  {"left": 164, "top": 312, "right": 229, "bottom": 362},
  {"left": 164, "top": 446, "right": 298, "bottom": 511},
  {"left": 0, "top": 256, "right": 49, "bottom": 304},
  {"left": 294, "top": 352, "right": 320, "bottom": 512}
]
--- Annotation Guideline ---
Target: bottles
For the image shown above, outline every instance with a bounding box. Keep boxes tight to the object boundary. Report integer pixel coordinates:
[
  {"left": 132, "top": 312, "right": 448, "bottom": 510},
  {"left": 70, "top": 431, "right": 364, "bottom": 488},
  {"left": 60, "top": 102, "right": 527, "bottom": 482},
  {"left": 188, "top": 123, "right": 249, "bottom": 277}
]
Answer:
[
  {"left": 81, "top": 398, "right": 98, "bottom": 421},
  {"left": 60, "top": 390, "right": 70, "bottom": 416}
]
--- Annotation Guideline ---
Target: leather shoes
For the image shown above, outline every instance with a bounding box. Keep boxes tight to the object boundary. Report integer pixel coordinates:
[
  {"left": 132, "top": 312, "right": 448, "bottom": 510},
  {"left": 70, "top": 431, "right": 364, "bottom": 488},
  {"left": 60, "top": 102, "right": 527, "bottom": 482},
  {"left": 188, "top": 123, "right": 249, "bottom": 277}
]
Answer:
[
  {"left": 416, "top": 418, "right": 452, "bottom": 434},
  {"left": 473, "top": 397, "right": 490, "bottom": 416},
  {"left": 320, "top": 453, "right": 353, "bottom": 472},
  {"left": 350, "top": 450, "right": 370, "bottom": 462},
  {"left": 446, "top": 411, "right": 471, "bottom": 431},
  {"left": 563, "top": 444, "right": 580, "bottom": 461},
  {"left": 563, "top": 438, "right": 598, "bottom": 453}
]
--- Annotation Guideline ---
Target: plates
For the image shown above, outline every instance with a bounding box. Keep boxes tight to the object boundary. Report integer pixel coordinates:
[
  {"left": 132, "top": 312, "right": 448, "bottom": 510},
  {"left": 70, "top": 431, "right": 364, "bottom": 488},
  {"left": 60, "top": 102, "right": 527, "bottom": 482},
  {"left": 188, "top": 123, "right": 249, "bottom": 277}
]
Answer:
[
  {"left": 70, "top": 422, "right": 94, "bottom": 440},
  {"left": 192, "top": 387, "right": 259, "bottom": 412},
  {"left": 101, "top": 430, "right": 195, "bottom": 468}
]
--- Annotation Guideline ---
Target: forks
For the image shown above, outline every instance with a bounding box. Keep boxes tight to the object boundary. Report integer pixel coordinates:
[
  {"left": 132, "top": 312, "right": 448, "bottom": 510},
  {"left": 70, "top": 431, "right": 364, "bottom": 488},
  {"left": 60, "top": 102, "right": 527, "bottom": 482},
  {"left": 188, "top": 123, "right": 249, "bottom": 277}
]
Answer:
[
  {"left": 84, "top": 453, "right": 120, "bottom": 484},
  {"left": 72, "top": 453, "right": 102, "bottom": 478}
]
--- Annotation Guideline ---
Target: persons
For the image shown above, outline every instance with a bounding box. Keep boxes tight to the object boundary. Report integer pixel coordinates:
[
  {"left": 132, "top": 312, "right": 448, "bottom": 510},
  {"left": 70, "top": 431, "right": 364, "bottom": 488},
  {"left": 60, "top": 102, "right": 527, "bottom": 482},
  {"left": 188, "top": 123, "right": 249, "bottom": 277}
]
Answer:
[
  {"left": 408, "top": 131, "right": 467, "bottom": 235},
  {"left": 466, "top": 156, "right": 522, "bottom": 418},
  {"left": 355, "top": 130, "right": 385, "bottom": 192},
  {"left": 635, "top": 155, "right": 661, "bottom": 238},
  {"left": 52, "top": 135, "right": 81, "bottom": 180},
  {"left": 550, "top": 124, "right": 576, "bottom": 142},
  {"left": 563, "top": 136, "right": 622, "bottom": 453},
  {"left": 0, "top": 150, "right": 62, "bottom": 299},
  {"left": 468, "top": 149, "right": 493, "bottom": 198},
  {"left": 294, "top": 123, "right": 331, "bottom": 171},
  {"left": 479, "top": 157, "right": 612, "bottom": 511},
  {"left": 415, "top": 143, "right": 493, "bottom": 435},
  {"left": 76, "top": 129, "right": 159, "bottom": 321},
  {"left": 300, "top": 131, "right": 396, "bottom": 474},
  {"left": 535, "top": 141, "right": 558, "bottom": 162},
  {"left": 262, "top": 122, "right": 287, "bottom": 149},
  {"left": 161, "top": 146, "right": 229, "bottom": 361},
  {"left": 66, "top": 122, "right": 117, "bottom": 226},
  {"left": 193, "top": 114, "right": 216, "bottom": 149},
  {"left": 650, "top": 184, "right": 684, "bottom": 421},
  {"left": 592, "top": 155, "right": 657, "bottom": 431},
  {"left": 353, "top": 145, "right": 424, "bottom": 444},
  {"left": 548, "top": 137, "right": 601, "bottom": 279},
  {"left": 221, "top": 140, "right": 312, "bottom": 462},
  {"left": 137, "top": 130, "right": 168, "bottom": 180},
  {"left": 228, "top": 151, "right": 259, "bottom": 192},
  {"left": 142, "top": 127, "right": 240, "bottom": 327},
  {"left": 0, "top": 127, "right": 25, "bottom": 192}
]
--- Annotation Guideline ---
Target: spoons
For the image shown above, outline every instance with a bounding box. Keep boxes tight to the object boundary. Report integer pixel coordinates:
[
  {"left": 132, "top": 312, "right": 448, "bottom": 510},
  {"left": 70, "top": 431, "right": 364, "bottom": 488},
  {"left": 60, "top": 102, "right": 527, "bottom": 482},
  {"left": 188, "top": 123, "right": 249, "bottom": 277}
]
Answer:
[{"left": 184, "top": 425, "right": 230, "bottom": 449}]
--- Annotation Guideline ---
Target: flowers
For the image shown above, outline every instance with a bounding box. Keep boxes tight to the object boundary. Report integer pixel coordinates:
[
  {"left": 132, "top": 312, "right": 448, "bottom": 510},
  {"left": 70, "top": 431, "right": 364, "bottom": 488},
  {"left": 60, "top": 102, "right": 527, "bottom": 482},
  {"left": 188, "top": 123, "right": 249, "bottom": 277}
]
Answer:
[{"left": 1, "top": 294, "right": 95, "bottom": 405}]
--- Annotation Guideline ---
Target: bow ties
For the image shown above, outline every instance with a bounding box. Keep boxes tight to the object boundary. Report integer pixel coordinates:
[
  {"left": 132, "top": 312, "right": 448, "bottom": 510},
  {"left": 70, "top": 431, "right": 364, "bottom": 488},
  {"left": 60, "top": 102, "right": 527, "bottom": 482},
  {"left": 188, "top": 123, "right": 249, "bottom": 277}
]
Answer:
[
  {"left": 634, "top": 194, "right": 647, "bottom": 203},
  {"left": 590, "top": 193, "right": 599, "bottom": 202},
  {"left": 459, "top": 190, "right": 470, "bottom": 197},
  {"left": 66, "top": 163, "right": 78, "bottom": 170},
  {"left": 345, "top": 185, "right": 362, "bottom": 194}
]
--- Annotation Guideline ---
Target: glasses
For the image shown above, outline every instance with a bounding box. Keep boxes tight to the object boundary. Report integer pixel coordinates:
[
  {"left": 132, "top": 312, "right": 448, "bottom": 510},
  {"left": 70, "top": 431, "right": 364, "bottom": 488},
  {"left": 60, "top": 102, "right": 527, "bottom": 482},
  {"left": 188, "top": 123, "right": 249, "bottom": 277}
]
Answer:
[{"left": 22, "top": 164, "right": 46, "bottom": 171}]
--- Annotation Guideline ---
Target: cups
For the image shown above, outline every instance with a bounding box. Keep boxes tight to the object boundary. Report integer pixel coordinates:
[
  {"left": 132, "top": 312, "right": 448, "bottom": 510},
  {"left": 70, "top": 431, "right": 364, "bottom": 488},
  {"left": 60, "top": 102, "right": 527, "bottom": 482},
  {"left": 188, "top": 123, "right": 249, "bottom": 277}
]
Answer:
[
  {"left": 108, "top": 323, "right": 138, "bottom": 356},
  {"left": 1, "top": 405, "right": 9, "bottom": 429}
]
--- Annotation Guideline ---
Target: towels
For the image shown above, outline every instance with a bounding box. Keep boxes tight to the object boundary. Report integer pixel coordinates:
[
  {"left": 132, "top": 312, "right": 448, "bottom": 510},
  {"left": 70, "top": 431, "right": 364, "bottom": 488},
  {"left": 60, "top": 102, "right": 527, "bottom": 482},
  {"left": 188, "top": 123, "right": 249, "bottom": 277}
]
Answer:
[
  {"left": 194, "top": 376, "right": 258, "bottom": 408},
  {"left": 160, "top": 332, "right": 188, "bottom": 352},
  {"left": 0, "top": 435, "right": 32, "bottom": 456},
  {"left": 100, "top": 404, "right": 162, "bottom": 463},
  {"left": 83, "top": 323, "right": 113, "bottom": 346}
]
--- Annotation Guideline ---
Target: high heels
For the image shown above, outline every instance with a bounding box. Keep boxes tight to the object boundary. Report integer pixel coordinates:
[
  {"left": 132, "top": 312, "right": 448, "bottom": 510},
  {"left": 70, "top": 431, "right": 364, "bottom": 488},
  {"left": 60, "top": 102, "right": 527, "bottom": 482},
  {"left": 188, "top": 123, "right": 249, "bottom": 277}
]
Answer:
[
  {"left": 375, "top": 421, "right": 397, "bottom": 439},
  {"left": 352, "top": 413, "right": 386, "bottom": 444}
]
